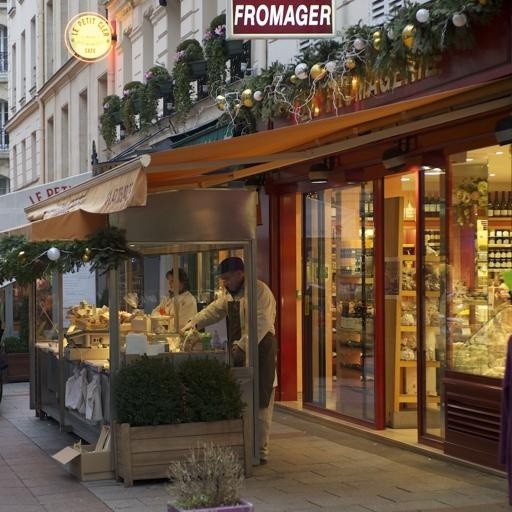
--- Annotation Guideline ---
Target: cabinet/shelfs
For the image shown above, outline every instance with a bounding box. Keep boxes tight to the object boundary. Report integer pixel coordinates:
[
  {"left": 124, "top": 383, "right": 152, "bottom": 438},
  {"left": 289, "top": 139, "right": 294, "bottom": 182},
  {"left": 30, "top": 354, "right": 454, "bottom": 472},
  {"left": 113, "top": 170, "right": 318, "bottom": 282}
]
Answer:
[
  {"left": 474, "top": 216, "right": 512, "bottom": 294},
  {"left": 330, "top": 218, "right": 374, "bottom": 385},
  {"left": 398, "top": 200, "right": 446, "bottom": 418}
]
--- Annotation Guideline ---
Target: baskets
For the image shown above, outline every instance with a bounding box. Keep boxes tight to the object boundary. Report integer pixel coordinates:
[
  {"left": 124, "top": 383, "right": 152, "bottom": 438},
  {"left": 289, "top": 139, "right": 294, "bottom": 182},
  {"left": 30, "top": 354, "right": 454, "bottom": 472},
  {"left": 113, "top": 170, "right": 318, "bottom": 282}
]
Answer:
[{"left": 341, "top": 316, "right": 369, "bottom": 329}]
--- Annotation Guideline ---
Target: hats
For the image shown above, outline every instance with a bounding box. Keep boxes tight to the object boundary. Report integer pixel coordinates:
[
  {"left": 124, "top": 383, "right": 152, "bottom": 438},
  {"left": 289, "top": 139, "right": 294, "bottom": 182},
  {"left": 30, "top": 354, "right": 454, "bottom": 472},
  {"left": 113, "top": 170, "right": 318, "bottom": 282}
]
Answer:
[{"left": 210, "top": 257, "right": 244, "bottom": 275}]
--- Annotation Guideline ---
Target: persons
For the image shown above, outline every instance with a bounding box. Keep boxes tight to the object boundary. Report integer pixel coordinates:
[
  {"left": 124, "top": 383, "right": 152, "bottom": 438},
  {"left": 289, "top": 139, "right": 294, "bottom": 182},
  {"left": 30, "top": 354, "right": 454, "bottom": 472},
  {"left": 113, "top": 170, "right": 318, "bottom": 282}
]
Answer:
[
  {"left": 150, "top": 267, "right": 198, "bottom": 332},
  {"left": 181, "top": 257, "right": 277, "bottom": 465}
]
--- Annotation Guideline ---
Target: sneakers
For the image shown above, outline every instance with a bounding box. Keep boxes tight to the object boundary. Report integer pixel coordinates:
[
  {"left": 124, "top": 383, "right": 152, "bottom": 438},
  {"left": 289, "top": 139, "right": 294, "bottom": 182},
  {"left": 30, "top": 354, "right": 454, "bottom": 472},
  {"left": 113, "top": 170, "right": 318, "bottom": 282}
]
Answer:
[{"left": 252, "top": 459, "right": 268, "bottom": 467}]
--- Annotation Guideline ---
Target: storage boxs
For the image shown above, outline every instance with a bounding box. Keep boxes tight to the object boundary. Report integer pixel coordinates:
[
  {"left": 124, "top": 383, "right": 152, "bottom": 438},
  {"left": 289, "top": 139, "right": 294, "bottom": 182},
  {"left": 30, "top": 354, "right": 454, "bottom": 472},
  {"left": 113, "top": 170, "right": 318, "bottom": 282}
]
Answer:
[{"left": 51, "top": 444, "right": 114, "bottom": 481}]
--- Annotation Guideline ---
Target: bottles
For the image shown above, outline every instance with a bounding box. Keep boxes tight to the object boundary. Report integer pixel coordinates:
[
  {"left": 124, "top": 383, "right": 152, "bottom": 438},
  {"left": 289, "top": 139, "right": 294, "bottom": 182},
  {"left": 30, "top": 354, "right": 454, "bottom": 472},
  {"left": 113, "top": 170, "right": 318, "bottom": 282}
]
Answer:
[
  {"left": 488, "top": 190, "right": 512, "bottom": 217},
  {"left": 363, "top": 192, "right": 373, "bottom": 217},
  {"left": 331, "top": 197, "right": 337, "bottom": 217},
  {"left": 424, "top": 192, "right": 441, "bottom": 217}
]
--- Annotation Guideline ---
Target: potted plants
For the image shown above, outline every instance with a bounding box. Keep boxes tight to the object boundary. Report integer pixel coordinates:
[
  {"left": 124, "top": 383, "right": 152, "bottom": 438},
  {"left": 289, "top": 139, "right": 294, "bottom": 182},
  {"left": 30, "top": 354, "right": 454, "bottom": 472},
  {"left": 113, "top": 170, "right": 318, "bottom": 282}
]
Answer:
[
  {"left": 115, "top": 353, "right": 254, "bottom": 488},
  {"left": 167, "top": 440, "right": 253, "bottom": 512},
  {"left": 0, "top": 336, "right": 31, "bottom": 382}
]
